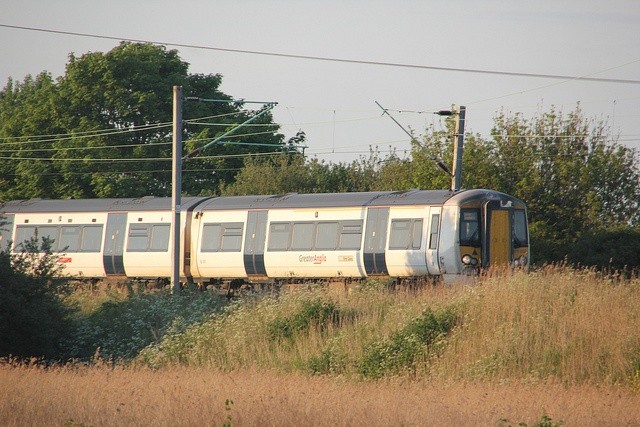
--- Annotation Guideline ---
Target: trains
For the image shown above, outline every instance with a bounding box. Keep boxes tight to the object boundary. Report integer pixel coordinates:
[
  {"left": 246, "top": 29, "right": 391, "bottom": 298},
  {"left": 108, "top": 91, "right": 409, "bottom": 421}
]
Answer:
[{"left": 0, "top": 189, "right": 530, "bottom": 301}]
